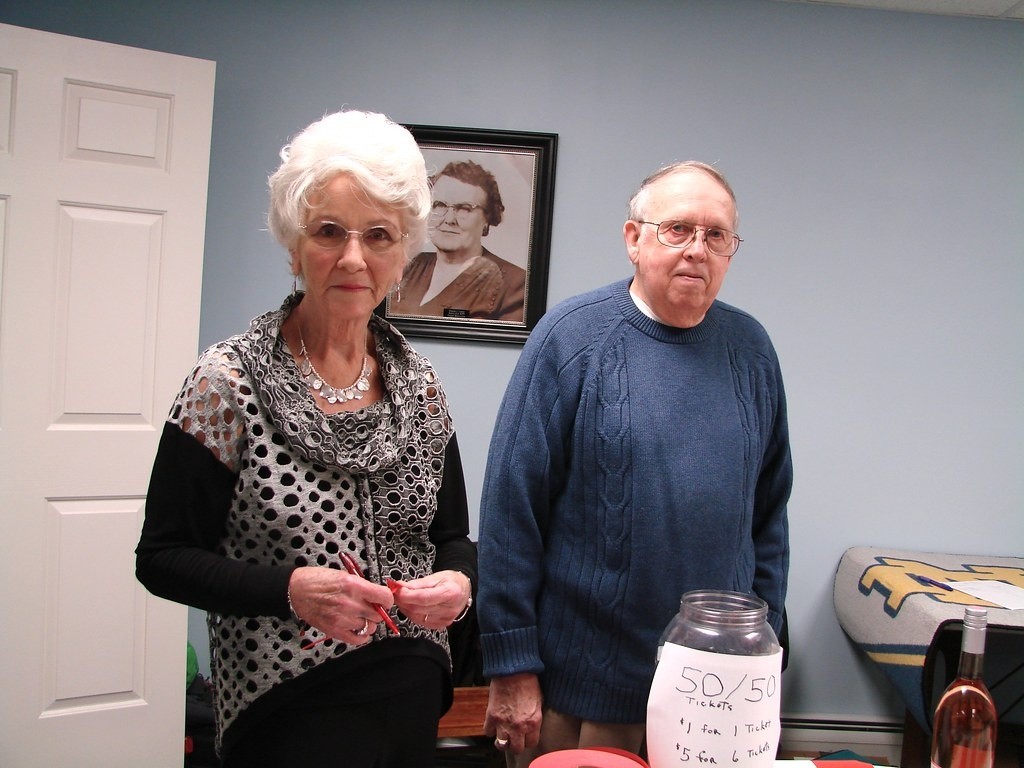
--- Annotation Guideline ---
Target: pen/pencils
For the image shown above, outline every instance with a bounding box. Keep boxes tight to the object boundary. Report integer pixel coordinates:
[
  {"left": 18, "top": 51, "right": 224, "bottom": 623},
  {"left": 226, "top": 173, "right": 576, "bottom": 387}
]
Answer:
[{"left": 339, "top": 550, "right": 401, "bottom": 638}]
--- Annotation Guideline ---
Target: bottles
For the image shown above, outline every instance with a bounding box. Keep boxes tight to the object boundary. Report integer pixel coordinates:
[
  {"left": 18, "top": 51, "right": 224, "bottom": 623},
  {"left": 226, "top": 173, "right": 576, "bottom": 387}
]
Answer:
[
  {"left": 930, "top": 606, "right": 998, "bottom": 768},
  {"left": 654, "top": 589, "right": 779, "bottom": 677}
]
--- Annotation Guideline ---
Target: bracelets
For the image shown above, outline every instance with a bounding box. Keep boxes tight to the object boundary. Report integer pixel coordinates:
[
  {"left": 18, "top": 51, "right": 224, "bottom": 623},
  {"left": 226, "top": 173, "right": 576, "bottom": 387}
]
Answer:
[
  {"left": 453, "top": 571, "right": 472, "bottom": 622},
  {"left": 287, "top": 586, "right": 294, "bottom": 613}
]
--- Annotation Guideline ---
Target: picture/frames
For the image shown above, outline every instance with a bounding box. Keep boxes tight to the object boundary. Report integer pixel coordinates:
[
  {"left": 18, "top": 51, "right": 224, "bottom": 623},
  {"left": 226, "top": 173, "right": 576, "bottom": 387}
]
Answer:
[{"left": 373, "top": 123, "right": 559, "bottom": 344}]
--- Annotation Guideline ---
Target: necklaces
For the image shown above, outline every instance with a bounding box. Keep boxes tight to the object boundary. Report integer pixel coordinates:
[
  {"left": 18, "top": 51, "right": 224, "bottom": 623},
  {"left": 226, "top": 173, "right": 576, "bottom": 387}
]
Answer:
[{"left": 296, "top": 318, "right": 371, "bottom": 403}]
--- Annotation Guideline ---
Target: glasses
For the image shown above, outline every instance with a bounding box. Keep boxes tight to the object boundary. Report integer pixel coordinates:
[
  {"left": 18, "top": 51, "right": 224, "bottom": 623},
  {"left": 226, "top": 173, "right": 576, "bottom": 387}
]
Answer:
[
  {"left": 300, "top": 221, "right": 409, "bottom": 253},
  {"left": 431, "top": 200, "right": 491, "bottom": 217},
  {"left": 638, "top": 219, "right": 744, "bottom": 258}
]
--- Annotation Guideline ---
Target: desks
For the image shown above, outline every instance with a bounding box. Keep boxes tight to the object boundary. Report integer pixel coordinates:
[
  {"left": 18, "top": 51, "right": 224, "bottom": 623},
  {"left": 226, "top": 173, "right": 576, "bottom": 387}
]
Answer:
[{"left": 833, "top": 547, "right": 1024, "bottom": 768}]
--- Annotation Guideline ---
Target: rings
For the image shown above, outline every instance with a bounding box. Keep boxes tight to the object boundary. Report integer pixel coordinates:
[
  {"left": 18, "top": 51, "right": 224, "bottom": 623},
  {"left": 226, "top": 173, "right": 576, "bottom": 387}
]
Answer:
[
  {"left": 424, "top": 614, "right": 428, "bottom": 622},
  {"left": 496, "top": 737, "right": 508, "bottom": 746},
  {"left": 357, "top": 619, "right": 368, "bottom": 635}
]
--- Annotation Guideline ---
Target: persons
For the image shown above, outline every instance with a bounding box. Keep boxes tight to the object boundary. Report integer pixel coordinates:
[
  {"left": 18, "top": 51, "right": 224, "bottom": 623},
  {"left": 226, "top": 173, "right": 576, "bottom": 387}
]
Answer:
[
  {"left": 477, "top": 162, "right": 793, "bottom": 768},
  {"left": 391, "top": 161, "right": 525, "bottom": 324},
  {"left": 136, "top": 111, "right": 479, "bottom": 768}
]
